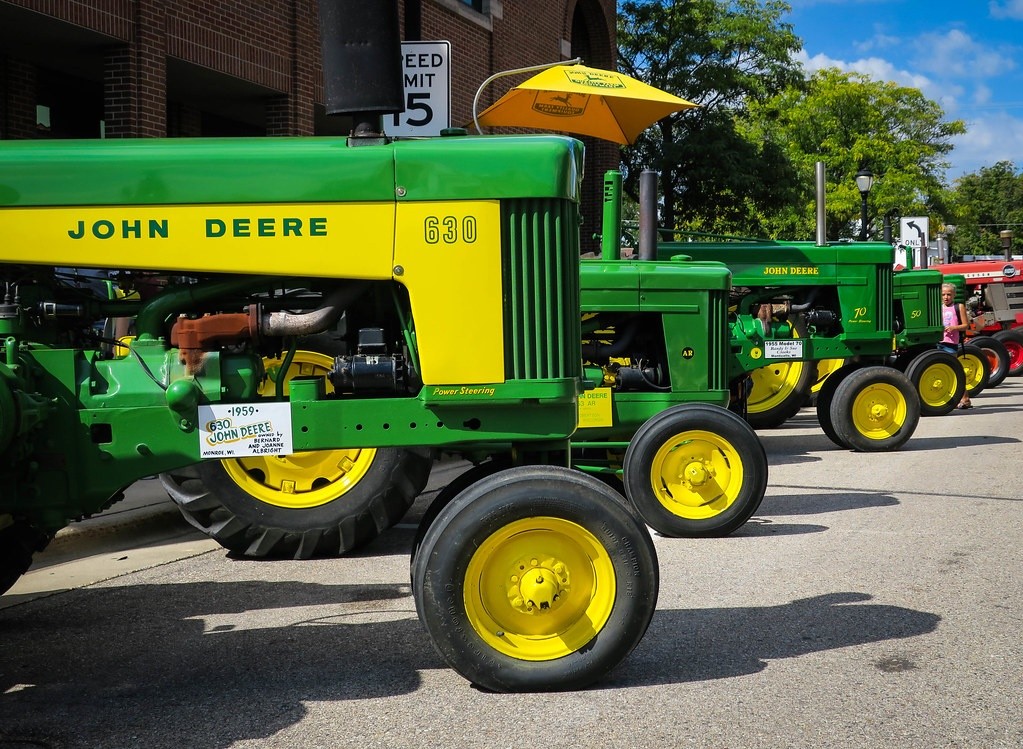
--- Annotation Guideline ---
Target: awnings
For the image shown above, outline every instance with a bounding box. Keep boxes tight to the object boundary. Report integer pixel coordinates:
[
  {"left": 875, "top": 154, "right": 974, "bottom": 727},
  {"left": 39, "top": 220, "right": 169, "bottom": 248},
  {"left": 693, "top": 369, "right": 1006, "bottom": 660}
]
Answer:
[{"left": 463, "top": 57, "right": 702, "bottom": 146}]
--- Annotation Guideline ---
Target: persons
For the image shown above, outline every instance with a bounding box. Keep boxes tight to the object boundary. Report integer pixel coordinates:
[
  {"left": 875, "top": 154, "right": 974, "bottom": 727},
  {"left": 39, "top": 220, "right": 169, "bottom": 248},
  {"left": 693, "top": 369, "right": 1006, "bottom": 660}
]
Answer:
[{"left": 941, "top": 283, "right": 971, "bottom": 410}]
